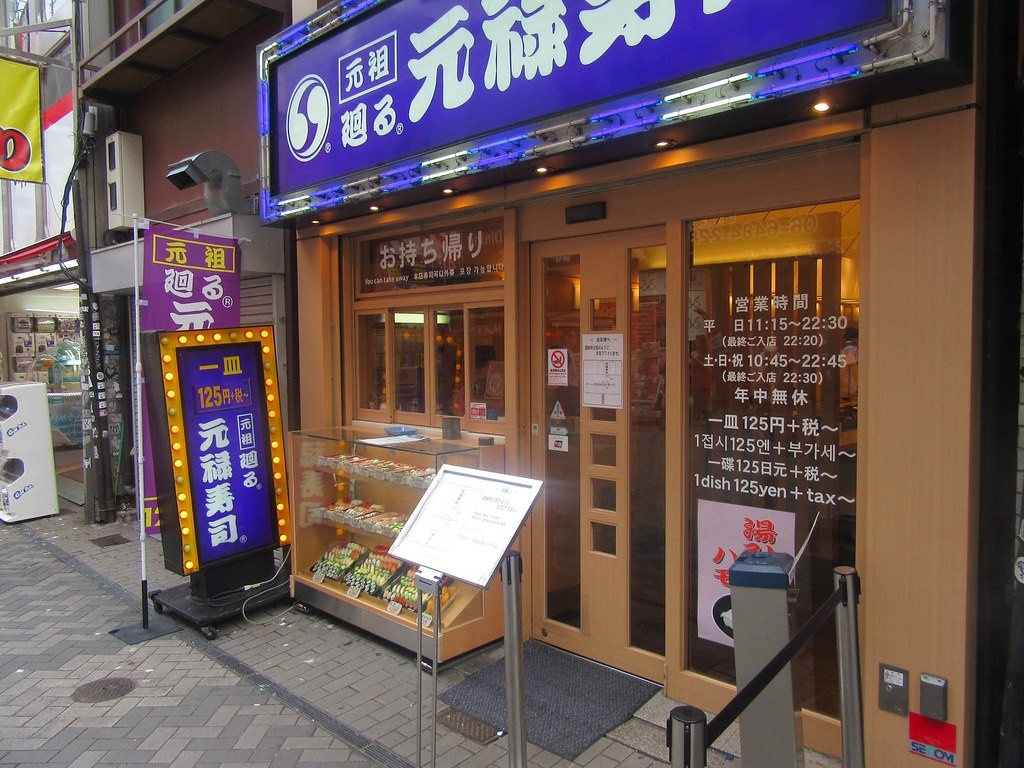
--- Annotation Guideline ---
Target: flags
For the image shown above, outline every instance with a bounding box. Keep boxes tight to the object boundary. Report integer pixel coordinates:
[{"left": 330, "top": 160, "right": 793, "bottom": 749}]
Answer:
[{"left": 0, "top": 55, "right": 45, "bottom": 184}]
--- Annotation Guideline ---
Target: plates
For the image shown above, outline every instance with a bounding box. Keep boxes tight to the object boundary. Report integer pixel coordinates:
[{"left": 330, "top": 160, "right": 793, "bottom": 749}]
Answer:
[
  {"left": 384, "top": 427, "right": 418, "bottom": 435},
  {"left": 712, "top": 595, "right": 733, "bottom": 639}
]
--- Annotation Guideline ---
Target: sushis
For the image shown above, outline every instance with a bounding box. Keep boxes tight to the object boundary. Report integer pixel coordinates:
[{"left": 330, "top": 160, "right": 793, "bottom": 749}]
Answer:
[{"left": 312, "top": 541, "right": 429, "bottom": 610}]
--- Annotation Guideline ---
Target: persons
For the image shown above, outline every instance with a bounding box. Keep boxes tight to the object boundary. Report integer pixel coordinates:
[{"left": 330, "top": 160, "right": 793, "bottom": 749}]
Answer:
[
  {"left": 546, "top": 344, "right": 572, "bottom": 415},
  {"left": 842, "top": 327, "right": 858, "bottom": 395},
  {"left": 649, "top": 339, "right": 710, "bottom": 434}
]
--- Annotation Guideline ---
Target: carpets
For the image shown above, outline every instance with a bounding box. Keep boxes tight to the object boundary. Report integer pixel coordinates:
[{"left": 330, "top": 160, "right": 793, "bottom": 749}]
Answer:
[{"left": 436, "top": 637, "right": 664, "bottom": 762}]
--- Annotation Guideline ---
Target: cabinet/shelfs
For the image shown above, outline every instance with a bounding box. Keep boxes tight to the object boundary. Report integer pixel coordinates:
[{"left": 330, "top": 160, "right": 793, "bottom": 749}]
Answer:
[
  {"left": 287, "top": 424, "right": 506, "bottom": 676},
  {"left": 0, "top": 380, "right": 60, "bottom": 525}
]
